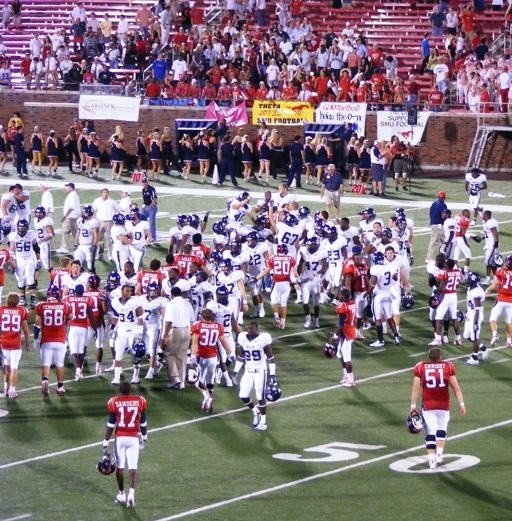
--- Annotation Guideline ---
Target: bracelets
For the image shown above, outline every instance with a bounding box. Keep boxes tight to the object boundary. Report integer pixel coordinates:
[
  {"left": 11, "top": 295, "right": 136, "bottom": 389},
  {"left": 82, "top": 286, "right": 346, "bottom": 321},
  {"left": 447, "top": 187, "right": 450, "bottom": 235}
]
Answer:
[
  {"left": 141, "top": 434, "right": 147, "bottom": 440},
  {"left": 103, "top": 439, "right": 109, "bottom": 447}
]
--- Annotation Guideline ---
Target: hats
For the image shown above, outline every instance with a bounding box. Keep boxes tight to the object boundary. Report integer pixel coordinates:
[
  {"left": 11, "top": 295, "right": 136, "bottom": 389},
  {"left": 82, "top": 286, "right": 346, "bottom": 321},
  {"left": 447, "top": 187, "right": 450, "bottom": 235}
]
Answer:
[
  {"left": 63, "top": 183, "right": 76, "bottom": 191},
  {"left": 351, "top": 246, "right": 364, "bottom": 256},
  {"left": 439, "top": 208, "right": 451, "bottom": 215},
  {"left": 215, "top": 286, "right": 230, "bottom": 300},
  {"left": 194, "top": 270, "right": 208, "bottom": 281},
  {"left": 140, "top": 177, "right": 148, "bottom": 184},
  {"left": 220, "top": 77, "right": 227, "bottom": 84},
  {"left": 437, "top": 191, "right": 446, "bottom": 200}
]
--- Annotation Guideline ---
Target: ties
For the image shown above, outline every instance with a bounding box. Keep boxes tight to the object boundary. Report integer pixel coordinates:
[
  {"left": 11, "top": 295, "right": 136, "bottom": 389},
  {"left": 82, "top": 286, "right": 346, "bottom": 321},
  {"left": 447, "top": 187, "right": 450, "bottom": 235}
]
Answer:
[{"left": 231, "top": 78, "right": 238, "bottom": 84}]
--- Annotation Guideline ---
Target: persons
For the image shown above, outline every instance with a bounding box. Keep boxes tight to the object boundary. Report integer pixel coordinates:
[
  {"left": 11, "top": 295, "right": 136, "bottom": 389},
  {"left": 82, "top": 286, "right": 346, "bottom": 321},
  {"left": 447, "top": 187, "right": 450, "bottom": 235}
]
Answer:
[
  {"left": 0, "top": 1, "right": 511, "bottom": 430},
  {"left": 409, "top": 348, "right": 467, "bottom": 470},
  {"left": 102, "top": 381, "right": 148, "bottom": 508}
]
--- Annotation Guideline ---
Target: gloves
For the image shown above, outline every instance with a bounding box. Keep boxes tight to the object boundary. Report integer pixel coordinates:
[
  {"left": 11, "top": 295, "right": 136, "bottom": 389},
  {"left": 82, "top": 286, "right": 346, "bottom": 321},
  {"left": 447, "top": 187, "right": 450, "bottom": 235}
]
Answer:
[
  {"left": 107, "top": 328, "right": 115, "bottom": 338},
  {"left": 137, "top": 243, "right": 148, "bottom": 250},
  {"left": 232, "top": 375, "right": 239, "bottom": 385},
  {"left": 227, "top": 352, "right": 237, "bottom": 363},
  {"left": 408, "top": 254, "right": 415, "bottom": 266},
  {"left": 204, "top": 210, "right": 212, "bottom": 219},
  {"left": 33, "top": 339, "right": 41, "bottom": 349},
  {"left": 190, "top": 357, "right": 198, "bottom": 367},
  {"left": 139, "top": 434, "right": 149, "bottom": 450},
  {"left": 102, "top": 446, "right": 111, "bottom": 458},
  {"left": 6, "top": 264, "right": 16, "bottom": 273},
  {"left": 73, "top": 244, "right": 79, "bottom": 251},
  {"left": 249, "top": 276, "right": 259, "bottom": 285},
  {"left": 439, "top": 243, "right": 448, "bottom": 255},
  {"left": 268, "top": 376, "right": 277, "bottom": 386},
  {"left": 494, "top": 252, "right": 499, "bottom": 259},
  {"left": 473, "top": 324, "right": 480, "bottom": 333},
  {"left": 37, "top": 236, "right": 47, "bottom": 244},
  {"left": 89, "top": 246, "right": 97, "bottom": 254},
  {"left": 35, "top": 262, "right": 42, "bottom": 271}
]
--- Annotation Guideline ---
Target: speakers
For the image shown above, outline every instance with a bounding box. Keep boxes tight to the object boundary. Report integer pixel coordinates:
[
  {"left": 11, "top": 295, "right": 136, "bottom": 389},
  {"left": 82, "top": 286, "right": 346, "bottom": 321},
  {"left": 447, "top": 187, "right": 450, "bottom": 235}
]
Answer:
[{"left": 407, "top": 108, "right": 417, "bottom": 126}]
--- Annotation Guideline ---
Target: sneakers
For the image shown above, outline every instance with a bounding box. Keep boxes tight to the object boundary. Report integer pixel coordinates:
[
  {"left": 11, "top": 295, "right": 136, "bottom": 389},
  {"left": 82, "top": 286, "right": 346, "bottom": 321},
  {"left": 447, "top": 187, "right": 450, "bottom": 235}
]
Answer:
[
  {"left": 395, "top": 187, "right": 399, "bottom": 191},
  {"left": 272, "top": 318, "right": 280, "bottom": 331},
  {"left": 153, "top": 363, "right": 164, "bottom": 376},
  {"left": 74, "top": 373, "right": 84, "bottom": 382},
  {"left": 3, "top": 389, "right": 9, "bottom": 398},
  {"left": 166, "top": 377, "right": 180, "bottom": 389},
  {"left": 370, "top": 338, "right": 385, "bottom": 347},
  {"left": 104, "top": 366, "right": 115, "bottom": 372},
  {"left": 294, "top": 290, "right": 341, "bottom": 307},
  {"left": 303, "top": 321, "right": 312, "bottom": 329},
  {"left": 201, "top": 397, "right": 215, "bottom": 416},
  {"left": 481, "top": 279, "right": 491, "bottom": 286},
  {"left": 255, "top": 309, "right": 266, "bottom": 319},
  {"left": 428, "top": 338, "right": 442, "bottom": 346},
  {"left": 237, "top": 318, "right": 245, "bottom": 325},
  {"left": 490, "top": 278, "right": 497, "bottom": 284},
  {"left": 362, "top": 322, "right": 372, "bottom": 331},
  {"left": 110, "top": 376, "right": 122, "bottom": 384},
  {"left": 115, "top": 492, "right": 127, "bottom": 504},
  {"left": 354, "top": 334, "right": 367, "bottom": 340},
  {"left": 215, "top": 368, "right": 223, "bottom": 386},
  {"left": 8, "top": 389, "right": 18, "bottom": 399},
  {"left": 174, "top": 381, "right": 186, "bottom": 391},
  {"left": 338, "top": 375, "right": 347, "bottom": 384},
  {"left": 41, "top": 381, "right": 50, "bottom": 397},
  {"left": 94, "top": 252, "right": 104, "bottom": 259},
  {"left": 95, "top": 368, "right": 105, "bottom": 375},
  {"left": 381, "top": 328, "right": 388, "bottom": 334},
  {"left": 255, "top": 422, "right": 267, "bottom": 431},
  {"left": 108, "top": 253, "right": 114, "bottom": 261},
  {"left": 126, "top": 495, "right": 135, "bottom": 508},
  {"left": 56, "top": 247, "right": 70, "bottom": 254},
  {"left": 129, "top": 375, "right": 141, "bottom": 385},
  {"left": 145, "top": 368, "right": 156, "bottom": 380},
  {"left": 427, "top": 455, "right": 444, "bottom": 469},
  {"left": 453, "top": 335, "right": 461, "bottom": 346},
  {"left": 379, "top": 192, "right": 387, "bottom": 198},
  {"left": 18, "top": 296, "right": 27, "bottom": 305},
  {"left": 374, "top": 191, "right": 379, "bottom": 196},
  {"left": 257, "top": 177, "right": 271, "bottom": 187},
  {"left": 394, "top": 335, "right": 401, "bottom": 346},
  {"left": 65, "top": 345, "right": 90, "bottom": 366},
  {"left": 402, "top": 185, "right": 408, "bottom": 192},
  {"left": 30, "top": 297, "right": 37, "bottom": 307},
  {"left": 155, "top": 350, "right": 168, "bottom": 367},
  {"left": 223, "top": 378, "right": 234, "bottom": 389},
  {"left": 491, "top": 335, "right": 500, "bottom": 347},
  {"left": 56, "top": 386, "right": 66, "bottom": 395},
  {"left": 344, "top": 381, "right": 356, "bottom": 388},
  {"left": 313, "top": 318, "right": 320, "bottom": 328},
  {"left": 442, "top": 335, "right": 449, "bottom": 344},
  {"left": 280, "top": 320, "right": 287, "bottom": 330},
  {"left": 251, "top": 411, "right": 262, "bottom": 426},
  {"left": 481, "top": 348, "right": 490, "bottom": 361},
  {"left": 466, "top": 357, "right": 480, "bottom": 366},
  {"left": 505, "top": 341, "right": 512, "bottom": 348}
]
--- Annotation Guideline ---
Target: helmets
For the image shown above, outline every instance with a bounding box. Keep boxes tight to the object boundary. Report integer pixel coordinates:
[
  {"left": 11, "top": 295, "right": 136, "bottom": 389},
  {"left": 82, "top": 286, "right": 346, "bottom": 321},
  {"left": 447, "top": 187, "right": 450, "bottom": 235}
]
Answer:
[
  {"left": 111, "top": 213, "right": 126, "bottom": 225},
  {"left": 209, "top": 251, "right": 223, "bottom": 263},
  {"left": 381, "top": 226, "right": 392, "bottom": 238},
  {"left": 306, "top": 236, "right": 320, "bottom": 250},
  {"left": 146, "top": 282, "right": 160, "bottom": 298},
  {"left": 188, "top": 214, "right": 200, "bottom": 227},
  {"left": 132, "top": 338, "right": 146, "bottom": 357},
  {"left": 212, "top": 221, "right": 226, "bottom": 234},
  {"left": 467, "top": 272, "right": 480, "bottom": 284},
  {"left": 221, "top": 258, "right": 234, "bottom": 271},
  {"left": 265, "top": 383, "right": 282, "bottom": 403},
  {"left": 504, "top": 255, "right": 512, "bottom": 269},
  {"left": 492, "top": 254, "right": 504, "bottom": 266},
  {"left": 394, "top": 208, "right": 407, "bottom": 218},
  {"left": 284, "top": 214, "right": 298, "bottom": 226},
  {"left": 245, "top": 231, "right": 258, "bottom": 242},
  {"left": 87, "top": 275, "right": 101, "bottom": 289},
  {"left": 313, "top": 211, "right": 338, "bottom": 241},
  {"left": 1, "top": 222, "right": 12, "bottom": 234},
  {"left": 371, "top": 251, "right": 385, "bottom": 265},
  {"left": 428, "top": 296, "right": 440, "bottom": 309},
  {"left": 323, "top": 344, "right": 337, "bottom": 358},
  {"left": 95, "top": 456, "right": 117, "bottom": 476},
  {"left": 130, "top": 209, "right": 139, "bottom": 220},
  {"left": 75, "top": 284, "right": 85, "bottom": 294},
  {"left": 176, "top": 215, "right": 188, "bottom": 228},
  {"left": 105, "top": 272, "right": 120, "bottom": 289},
  {"left": 470, "top": 188, "right": 478, "bottom": 196},
  {"left": 455, "top": 309, "right": 464, "bottom": 324},
  {"left": 46, "top": 284, "right": 60, "bottom": 298},
  {"left": 238, "top": 193, "right": 251, "bottom": 205},
  {"left": 185, "top": 364, "right": 201, "bottom": 386},
  {"left": 276, "top": 244, "right": 288, "bottom": 257},
  {"left": 406, "top": 413, "right": 426, "bottom": 434},
  {"left": 230, "top": 241, "right": 241, "bottom": 253},
  {"left": 35, "top": 205, "right": 47, "bottom": 218},
  {"left": 81, "top": 205, "right": 95, "bottom": 218},
  {"left": 396, "top": 219, "right": 407, "bottom": 228},
  {"left": 401, "top": 294, "right": 415, "bottom": 310},
  {"left": 299, "top": 205, "right": 311, "bottom": 217},
  {"left": 17, "top": 219, "right": 30, "bottom": 230},
  {"left": 363, "top": 208, "right": 376, "bottom": 222}
]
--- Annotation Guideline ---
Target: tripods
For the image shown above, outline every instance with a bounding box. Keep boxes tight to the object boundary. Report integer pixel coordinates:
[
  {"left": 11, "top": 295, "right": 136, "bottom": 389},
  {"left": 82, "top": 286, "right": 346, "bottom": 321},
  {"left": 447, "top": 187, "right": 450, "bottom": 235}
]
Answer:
[{"left": 407, "top": 126, "right": 425, "bottom": 177}]
